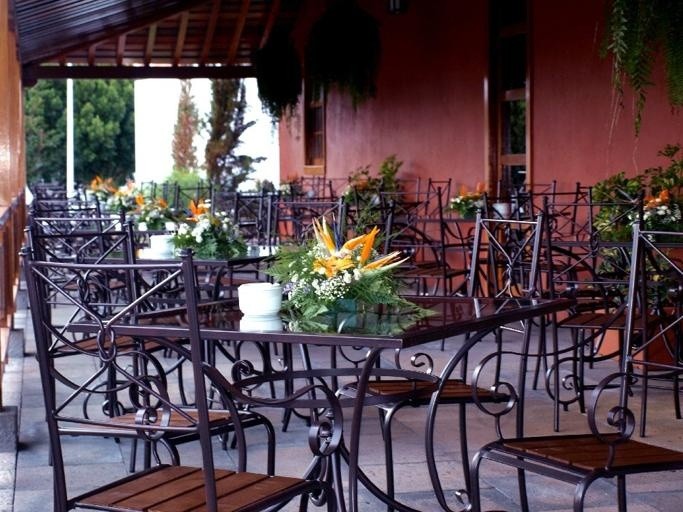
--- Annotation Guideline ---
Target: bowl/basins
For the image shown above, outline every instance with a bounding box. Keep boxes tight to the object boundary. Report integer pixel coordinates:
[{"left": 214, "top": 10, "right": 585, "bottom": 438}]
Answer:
[
  {"left": 238, "top": 316, "right": 283, "bottom": 334},
  {"left": 236, "top": 281, "right": 282, "bottom": 317},
  {"left": 491, "top": 204, "right": 510, "bottom": 217},
  {"left": 150, "top": 235, "right": 175, "bottom": 255}
]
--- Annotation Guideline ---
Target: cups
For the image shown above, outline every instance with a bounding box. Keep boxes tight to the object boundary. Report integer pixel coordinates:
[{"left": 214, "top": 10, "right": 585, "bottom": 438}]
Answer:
[{"left": 307, "top": 190, "right": 317, "bottom": 198}]
